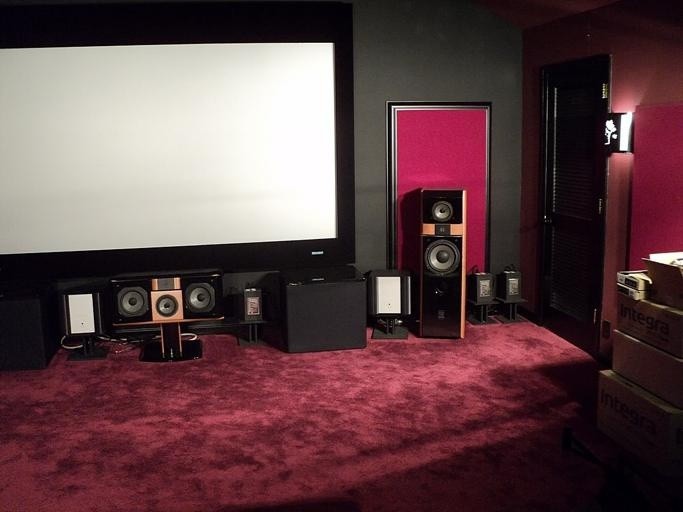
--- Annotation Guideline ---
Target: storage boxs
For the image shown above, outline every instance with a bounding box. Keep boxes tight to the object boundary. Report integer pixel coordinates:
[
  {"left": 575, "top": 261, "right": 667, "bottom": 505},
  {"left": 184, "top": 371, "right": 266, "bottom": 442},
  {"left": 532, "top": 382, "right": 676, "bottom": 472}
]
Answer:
[
  {"left": 279, "top": 265, "right": 366, "bottom": 353},
  {"left": 616, "top": 292, "right": 683, "bottom": 359},
  {"left": 598, "top": 369, "right": 683, "bottom": 462},
  {"left": 640, "top": 250, "right": 683, "bottom": 310},
  {"left": 612, "top": 329, "right": 683, "bottom": 409},
  {"left": 0, "top": 294, "right": 62, "bottom": 372}
]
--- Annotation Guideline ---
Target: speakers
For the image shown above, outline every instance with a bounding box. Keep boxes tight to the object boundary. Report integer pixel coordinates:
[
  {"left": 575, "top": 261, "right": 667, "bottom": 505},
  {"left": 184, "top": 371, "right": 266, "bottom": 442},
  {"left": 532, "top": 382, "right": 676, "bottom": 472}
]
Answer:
[
  {"left": 111, "top": 270, "right": 224, "bottom": 327},
  {"left": 419, "top": 188, "right": 466, "bottom": 340}
]
